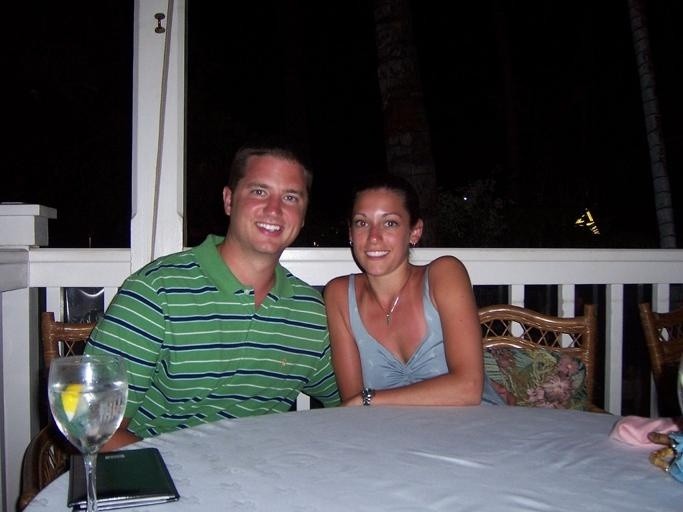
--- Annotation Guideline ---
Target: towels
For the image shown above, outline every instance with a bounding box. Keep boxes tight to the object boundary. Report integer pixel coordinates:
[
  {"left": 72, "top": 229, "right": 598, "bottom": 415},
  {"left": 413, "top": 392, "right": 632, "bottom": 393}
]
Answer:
[{"left": 609, "top": 415, "right": 679, "bottom": 447}]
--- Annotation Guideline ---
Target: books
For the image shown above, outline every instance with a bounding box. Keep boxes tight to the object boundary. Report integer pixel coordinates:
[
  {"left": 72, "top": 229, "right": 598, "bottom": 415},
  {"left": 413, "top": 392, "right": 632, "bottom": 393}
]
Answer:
[{"left": 66, "top": 447, "right": 180, "bottom": 512}]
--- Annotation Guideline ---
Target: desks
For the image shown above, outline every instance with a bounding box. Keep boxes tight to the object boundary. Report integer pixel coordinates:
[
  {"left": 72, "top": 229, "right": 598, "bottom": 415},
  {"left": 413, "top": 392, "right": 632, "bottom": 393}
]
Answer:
[{"left": 27, "top": 411, "right": 683, "bottom": 512}]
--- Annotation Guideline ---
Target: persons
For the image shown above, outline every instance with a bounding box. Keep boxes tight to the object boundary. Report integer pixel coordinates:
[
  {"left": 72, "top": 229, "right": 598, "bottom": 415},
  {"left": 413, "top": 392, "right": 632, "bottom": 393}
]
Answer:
[
  {"left": 321, "top": 172, "right": 485, "bottom": 407},
  {"left": 80, "top": 142, "right": 342, "bottom": 454}
]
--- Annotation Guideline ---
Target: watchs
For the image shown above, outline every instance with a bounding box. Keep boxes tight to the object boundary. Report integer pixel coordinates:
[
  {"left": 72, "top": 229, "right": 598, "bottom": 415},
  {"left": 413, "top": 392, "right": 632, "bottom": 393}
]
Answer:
[{"left": 361, "top": 386, "right": 375, "bottom": 405}]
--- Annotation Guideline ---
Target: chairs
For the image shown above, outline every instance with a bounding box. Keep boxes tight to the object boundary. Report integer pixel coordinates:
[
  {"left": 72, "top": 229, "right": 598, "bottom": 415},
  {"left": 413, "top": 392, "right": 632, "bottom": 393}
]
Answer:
[
  {"left": 637, "top": 302, "right": 683, "bottom": 416},
  {"left": 17, "top": 312, "right": 101, "bottom": 512},
  {"left": 477, "top": 304, "right": 616, "bottom": 415}
]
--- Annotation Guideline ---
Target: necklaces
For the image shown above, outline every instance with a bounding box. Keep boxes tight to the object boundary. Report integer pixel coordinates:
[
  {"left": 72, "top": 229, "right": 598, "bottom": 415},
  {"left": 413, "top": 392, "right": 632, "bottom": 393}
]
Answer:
[{"left": 364, "top": 270, "right": 412, "bottom": 325}]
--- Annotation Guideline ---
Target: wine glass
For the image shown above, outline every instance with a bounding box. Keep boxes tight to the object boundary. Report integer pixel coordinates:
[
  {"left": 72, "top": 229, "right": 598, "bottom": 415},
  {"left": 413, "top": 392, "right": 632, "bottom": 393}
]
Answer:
[{"left": 45, "top": 351, "right": 130, "bottom": 511}]
